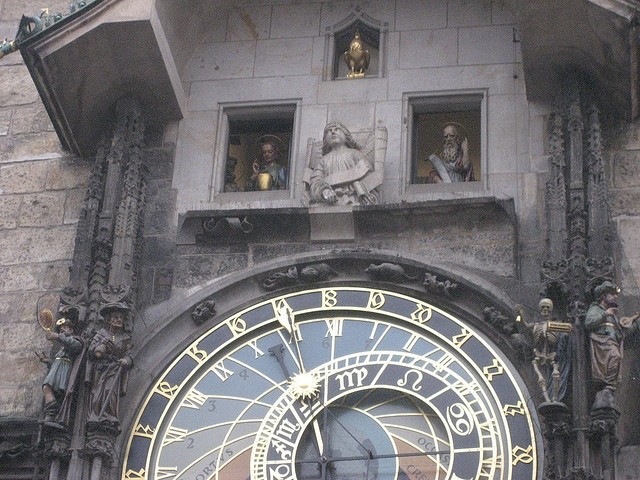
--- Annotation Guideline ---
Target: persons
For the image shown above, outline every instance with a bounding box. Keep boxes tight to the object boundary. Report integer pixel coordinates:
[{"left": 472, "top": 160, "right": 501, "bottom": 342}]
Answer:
[
  {"left": 34, "top": 311, "right": 82, "bottom": 425},
  {"left": 426, "top": 121, "right": 475, "bottom": 183},
  {"left": 84, "top": 301, "right": 137, "bottom": 427},
  {"left": 299, "top": 120, "right": 388, "bottom": 205},
  {"left": 513, "top": 297, "right": 573, "bottom": 403},
  {"left": 584, "top": 280, "right": 640, "bottom": 392},
  {"left": 245, "top": 131, "right": 286, "bottom": 191}
]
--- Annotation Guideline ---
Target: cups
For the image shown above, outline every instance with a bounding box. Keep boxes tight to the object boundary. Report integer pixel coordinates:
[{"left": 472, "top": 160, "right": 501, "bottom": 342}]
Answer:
[{"left": 257, "top": 172, "right": 273, "bottom": 191}]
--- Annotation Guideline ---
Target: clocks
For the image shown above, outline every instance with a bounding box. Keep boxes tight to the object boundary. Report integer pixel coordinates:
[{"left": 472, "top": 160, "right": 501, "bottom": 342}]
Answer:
[{"left": 116, "top": 281, "right": 545, "bottom": 480}]
